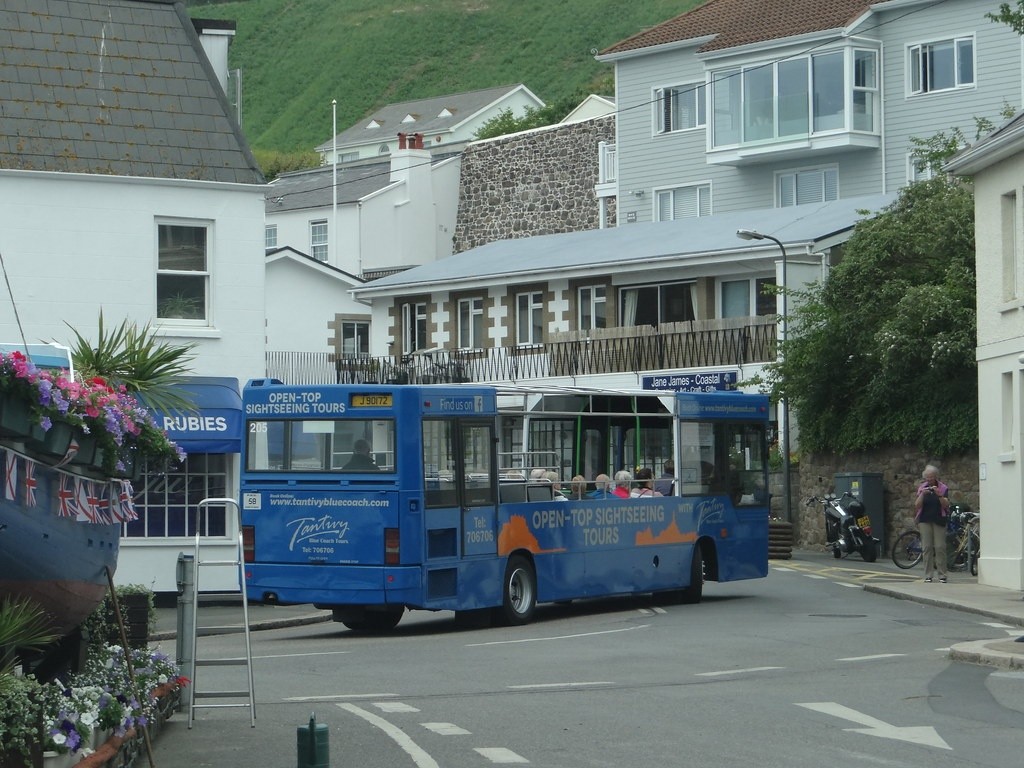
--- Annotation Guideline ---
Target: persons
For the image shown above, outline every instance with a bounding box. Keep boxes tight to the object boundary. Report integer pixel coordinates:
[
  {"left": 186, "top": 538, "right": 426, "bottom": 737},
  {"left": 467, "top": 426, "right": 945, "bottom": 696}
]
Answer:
[
  {"left": 567, "top": 475, "right": 593, "bottom": 500},
  {"left": 589, "top": 474, "right": 618, "bottom": 499},
  {"left": 655, "top": 460, "right": 674, "bottom": 496},
  {"left": 505, "top": 469, "right": 568, "bottom": 501},
  {"left": 342, "top": 439, "right": 380, "bottom": 470},
  {"left": 913, "top": 465, "right": 950, "bottom": 582},
  {"left": 612, "top": 471, "right": 632, "bottom": 498},
  {"left": 631, "top": 469, "right": 663, "bottom": 498}
]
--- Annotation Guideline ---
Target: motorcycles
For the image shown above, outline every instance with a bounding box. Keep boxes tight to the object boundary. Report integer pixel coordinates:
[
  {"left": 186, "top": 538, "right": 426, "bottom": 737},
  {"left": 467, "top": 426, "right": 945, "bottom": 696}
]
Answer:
[{"left": 805, "top": 492, "right": 881, "bottom": 562}]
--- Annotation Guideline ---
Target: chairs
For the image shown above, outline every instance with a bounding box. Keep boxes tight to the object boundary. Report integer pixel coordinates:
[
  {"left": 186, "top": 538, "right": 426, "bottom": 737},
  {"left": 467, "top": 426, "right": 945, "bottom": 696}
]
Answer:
[{"left": 428, "top": 473, "right": 553, "bottom": 503}]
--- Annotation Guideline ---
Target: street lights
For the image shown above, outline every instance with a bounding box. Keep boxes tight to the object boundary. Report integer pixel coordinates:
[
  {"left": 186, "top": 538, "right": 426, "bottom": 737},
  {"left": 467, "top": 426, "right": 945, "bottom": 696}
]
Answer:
[{"left": 736, "top": 229, "right": 793, "bottom": 524}]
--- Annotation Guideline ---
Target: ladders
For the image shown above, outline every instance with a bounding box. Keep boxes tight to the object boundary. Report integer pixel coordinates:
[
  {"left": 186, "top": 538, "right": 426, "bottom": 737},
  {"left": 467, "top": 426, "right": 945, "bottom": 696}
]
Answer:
[{"left": 187, "top": 497, "right": 258, "bottom": 729}]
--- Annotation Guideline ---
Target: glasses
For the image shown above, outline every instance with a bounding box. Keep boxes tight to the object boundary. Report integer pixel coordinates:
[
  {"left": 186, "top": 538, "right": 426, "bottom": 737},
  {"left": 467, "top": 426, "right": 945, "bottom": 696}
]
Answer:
[{"left": 551, "top": 479, "right": 561, "bottom": 484}]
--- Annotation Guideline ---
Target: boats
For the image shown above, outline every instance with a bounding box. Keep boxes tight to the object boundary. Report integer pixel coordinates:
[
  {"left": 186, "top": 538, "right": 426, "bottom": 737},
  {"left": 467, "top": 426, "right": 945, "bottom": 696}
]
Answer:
[{"left": 0, "top": 434, "right": 120, "bottom": 646}]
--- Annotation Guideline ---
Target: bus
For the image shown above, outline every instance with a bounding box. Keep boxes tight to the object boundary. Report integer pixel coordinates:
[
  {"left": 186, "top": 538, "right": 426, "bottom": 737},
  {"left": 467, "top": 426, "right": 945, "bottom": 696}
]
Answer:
[{"left": 237, "top": 379, "right": 771, "bottom": 630}]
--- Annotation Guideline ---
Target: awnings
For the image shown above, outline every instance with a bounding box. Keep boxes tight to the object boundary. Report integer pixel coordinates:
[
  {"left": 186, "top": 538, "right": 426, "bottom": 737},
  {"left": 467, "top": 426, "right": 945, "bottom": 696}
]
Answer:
[{"left": 133, "top": 376, "right": 242, "bottom": 454}]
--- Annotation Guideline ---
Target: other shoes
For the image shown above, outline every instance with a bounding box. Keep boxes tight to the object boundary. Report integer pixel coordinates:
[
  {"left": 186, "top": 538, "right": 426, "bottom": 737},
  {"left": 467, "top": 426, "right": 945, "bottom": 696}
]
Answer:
[
  {"left": 939, "top": 579, "right": 947, "bottom": 583},
  {"left": 926, "top": 577, "right": 932, "bottom": 582}
]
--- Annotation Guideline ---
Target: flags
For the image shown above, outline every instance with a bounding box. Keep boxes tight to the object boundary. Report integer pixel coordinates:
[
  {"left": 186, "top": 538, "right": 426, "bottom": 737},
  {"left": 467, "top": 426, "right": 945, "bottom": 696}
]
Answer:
[
  {"left": 73, "top": 477, "right": 112, "bottom": 525},
  {"left": 109, "top": 482, "right": 138, "bottom": 523},
  {"left": 58, "top": 473, "right": 79, "bottom": 516},
  {"left": 48, "top": 437, "right": 80, "bottom": 470},
  {"left": 0, "top": 450, "right": 19, "bottom": 502},
  {"left": 25, "top": 459, "right": 37, "bottom": 507}
]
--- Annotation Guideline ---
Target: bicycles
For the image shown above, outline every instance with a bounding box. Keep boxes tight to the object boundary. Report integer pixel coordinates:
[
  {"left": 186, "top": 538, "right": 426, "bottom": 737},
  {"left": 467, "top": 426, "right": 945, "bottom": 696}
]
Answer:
[{"left": 892, "top": 504, "right": 980, "bottom": 576}]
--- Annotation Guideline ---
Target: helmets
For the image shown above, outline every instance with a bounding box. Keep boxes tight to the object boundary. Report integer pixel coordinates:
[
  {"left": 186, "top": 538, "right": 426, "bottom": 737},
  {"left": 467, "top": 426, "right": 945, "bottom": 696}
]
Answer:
[{"left": 849, "top": 501, "right": 864, "bottom": 517}]
